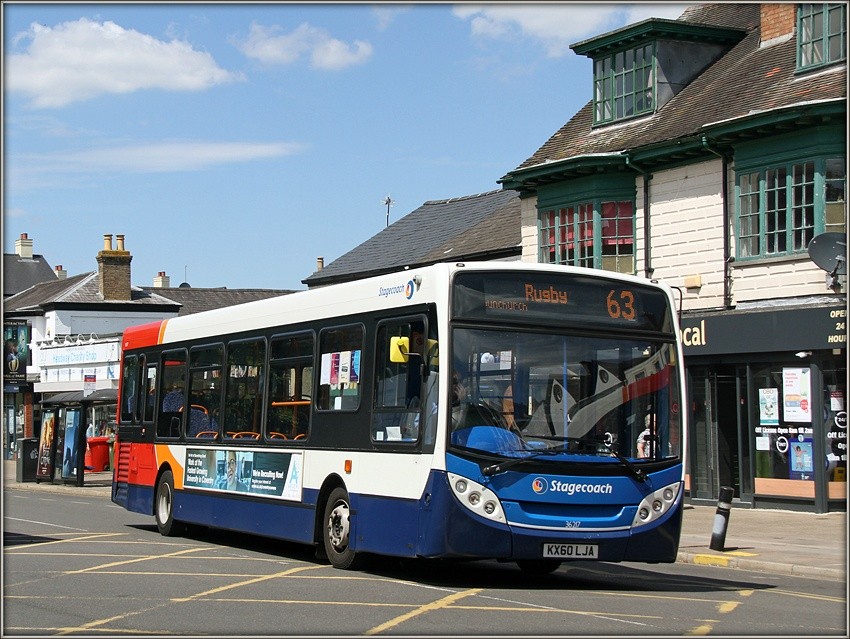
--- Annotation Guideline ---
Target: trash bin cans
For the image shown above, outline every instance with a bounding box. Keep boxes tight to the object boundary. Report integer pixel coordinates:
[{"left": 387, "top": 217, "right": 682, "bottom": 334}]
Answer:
[
  {"left": 16, "top": 437, "right": 39, "bottom": 482},
  {"left": 87, "top": 436, "right": 110, "bottom": 472},
  {"left": 107, "top": 434, "right": 116, "bottom": 472}
]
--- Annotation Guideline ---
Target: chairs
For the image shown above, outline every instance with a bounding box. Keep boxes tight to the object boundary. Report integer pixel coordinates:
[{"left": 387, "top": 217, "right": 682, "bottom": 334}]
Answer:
[{"left": 129, "top": 383, "right": 308, "bottom": 442}]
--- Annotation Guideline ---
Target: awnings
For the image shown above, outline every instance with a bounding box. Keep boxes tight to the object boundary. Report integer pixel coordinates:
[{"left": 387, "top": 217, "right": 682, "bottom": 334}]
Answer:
[
  {"left": 37, "top": 391, "right": 83, "bottom": 403},
  {"left": 80, "top": 389, "right": 119, "bottom": 401}
]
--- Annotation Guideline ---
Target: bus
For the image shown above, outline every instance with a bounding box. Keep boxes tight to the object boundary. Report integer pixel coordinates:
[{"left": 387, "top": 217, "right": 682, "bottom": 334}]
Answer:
[{"left": 110, "top": 260, "right": 687, "bottom": 570}]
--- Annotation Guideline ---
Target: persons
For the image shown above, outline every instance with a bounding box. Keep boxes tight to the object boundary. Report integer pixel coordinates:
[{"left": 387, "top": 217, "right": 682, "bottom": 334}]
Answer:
[
  {"left": 792, "top": 446, "right": 806, "bottom": 469},
  {"left": 418, "top": 337, "right": 474, "bottom": 445},
  {"left": 4, "top": 338, "right": 19, "bottom": 375},
  {"left": 85, "top": 419, "right": 116, "bottom": 472},
  {"left": 636, "top": 412, "right": 672, "bottom": 457},
  {"left": 43, "top": 416, "right": 53, "bottom": 448}
]
